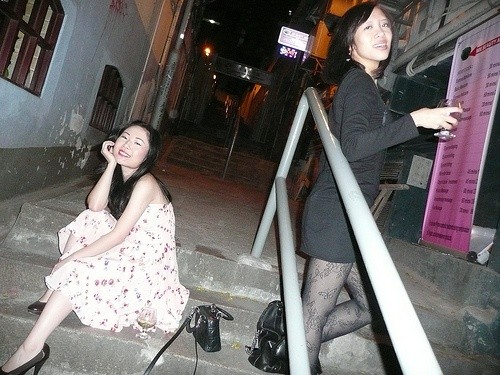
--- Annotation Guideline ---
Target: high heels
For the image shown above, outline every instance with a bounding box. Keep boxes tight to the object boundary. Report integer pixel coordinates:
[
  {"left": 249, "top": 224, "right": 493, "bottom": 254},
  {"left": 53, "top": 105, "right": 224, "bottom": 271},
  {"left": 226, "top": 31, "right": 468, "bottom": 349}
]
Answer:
[
  {"left": 0, "top": 344, "right": 50, "bottom": 375},
  {"left": 27, "top": 301, "right": 47, "bottom": 313}
]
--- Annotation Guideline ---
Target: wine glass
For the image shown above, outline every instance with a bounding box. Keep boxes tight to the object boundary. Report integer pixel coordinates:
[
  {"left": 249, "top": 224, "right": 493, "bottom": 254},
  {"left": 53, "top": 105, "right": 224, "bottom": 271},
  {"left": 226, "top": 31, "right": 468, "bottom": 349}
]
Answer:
[{"left": 433, "top": 99, "right": 462, "bottom": 139}]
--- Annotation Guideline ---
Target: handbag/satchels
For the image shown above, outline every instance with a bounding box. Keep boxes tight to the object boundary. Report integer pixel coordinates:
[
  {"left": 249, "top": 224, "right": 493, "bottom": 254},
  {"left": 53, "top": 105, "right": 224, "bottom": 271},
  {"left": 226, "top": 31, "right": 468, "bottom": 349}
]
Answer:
[
  {"left": 186, "top": 303, "right": 234, "bottom": 352},
  {"left": 245, "top": 302, "right": 323, "bottom": 375}
]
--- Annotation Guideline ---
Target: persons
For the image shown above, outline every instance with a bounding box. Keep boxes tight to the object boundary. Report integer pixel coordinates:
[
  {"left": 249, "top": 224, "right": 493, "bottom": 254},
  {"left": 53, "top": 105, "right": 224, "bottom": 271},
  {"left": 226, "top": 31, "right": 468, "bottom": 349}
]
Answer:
[
  {"left": 0, "top": 119, "right": 191, "bottom": 375},
  {"left": 296, "top": 1, "right": 463, "bottom": 375}
]
